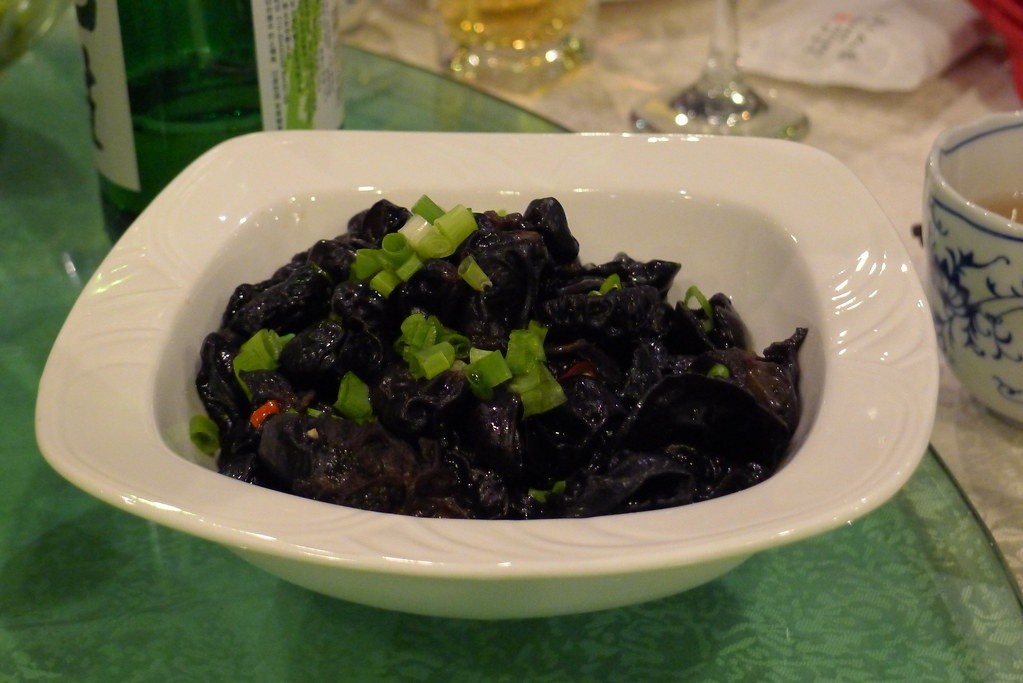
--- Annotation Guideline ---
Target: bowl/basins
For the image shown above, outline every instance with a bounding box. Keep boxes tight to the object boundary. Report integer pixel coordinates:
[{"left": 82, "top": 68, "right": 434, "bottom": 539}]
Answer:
[{"left": 31, "top": 126, "right": 941, "bottom": 622}]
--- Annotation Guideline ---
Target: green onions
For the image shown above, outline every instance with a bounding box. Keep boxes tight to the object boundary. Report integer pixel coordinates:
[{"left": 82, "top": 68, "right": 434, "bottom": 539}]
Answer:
[{"left": 188, "top": 198, "right": 732, "bottom": 503}]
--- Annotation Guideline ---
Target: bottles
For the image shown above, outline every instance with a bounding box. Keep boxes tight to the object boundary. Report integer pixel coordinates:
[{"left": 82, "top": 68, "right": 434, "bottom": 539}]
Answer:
[{"left": 70, "top": 0, "right": 347, "bottom": 250}]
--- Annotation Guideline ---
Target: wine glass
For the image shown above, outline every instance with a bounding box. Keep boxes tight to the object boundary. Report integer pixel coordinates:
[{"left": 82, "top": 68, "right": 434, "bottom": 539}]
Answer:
[{"left": 626, "top": 1, "right": 808, "bottom": 150}]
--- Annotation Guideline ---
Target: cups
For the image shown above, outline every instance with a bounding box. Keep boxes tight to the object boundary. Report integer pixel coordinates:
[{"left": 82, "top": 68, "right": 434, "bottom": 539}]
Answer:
[
  {"left": 922, "top": 107, "right": 1023, "bottom": 421},
  {"left": 421, "top": 1, "right": 603, "bottom": 85}
]
